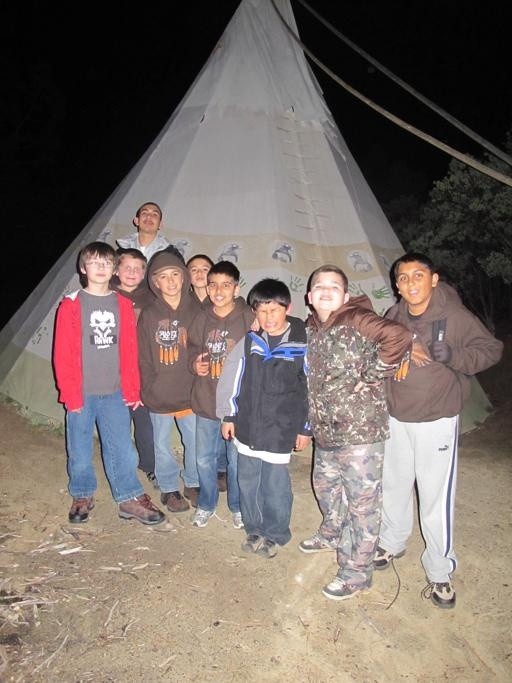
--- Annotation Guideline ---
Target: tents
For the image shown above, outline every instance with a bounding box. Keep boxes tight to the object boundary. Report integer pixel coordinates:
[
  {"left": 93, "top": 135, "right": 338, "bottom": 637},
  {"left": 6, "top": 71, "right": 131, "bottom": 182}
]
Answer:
[{"left": 0, "top": 1, "right": 498, "bottom": 457}]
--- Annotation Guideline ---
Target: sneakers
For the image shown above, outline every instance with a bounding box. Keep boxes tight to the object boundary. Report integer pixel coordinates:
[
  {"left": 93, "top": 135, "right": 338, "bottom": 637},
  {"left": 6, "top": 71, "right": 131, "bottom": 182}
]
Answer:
[
  {"left": 298, "top": 534, "right": 457, "bottom": 609},
  {"left": 68, "top": 470, "right": 281, "bottom": 561}
]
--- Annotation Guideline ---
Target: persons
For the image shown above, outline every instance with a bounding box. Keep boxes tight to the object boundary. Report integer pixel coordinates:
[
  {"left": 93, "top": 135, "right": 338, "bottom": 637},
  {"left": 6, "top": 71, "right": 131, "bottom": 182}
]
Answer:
[
  {"left": 114, "top": 202, "right": 185, "bottom": 263},
  {"left": 136, "top": 251, "right": 201, "bottom": 512},
  {"left": 298, "top": 265, "right": 413, "bottom": 600},
  {"left": 373, "top": 252, "right": 505, "bottom": 609},
  {"left": 186, "top": 260, "right": 255, "bottom": 529},
  {"left": 51, "top": 242, "right": 166, "bottom": 525},
  {"left": 215, "top": 278, "right": 313, "bottom": 558},
  {"left": 185, "top": 254, "right": 227, "bottom": 492},
  {"left": 109, "top": 248, "right": 159, "bottom": 489}
]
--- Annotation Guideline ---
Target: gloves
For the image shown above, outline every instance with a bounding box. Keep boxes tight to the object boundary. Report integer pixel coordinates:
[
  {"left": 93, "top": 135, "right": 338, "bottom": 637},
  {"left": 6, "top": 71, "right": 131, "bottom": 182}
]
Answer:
[{"left": 428, "top": 341, "right": 451, "bottom": 363}]
